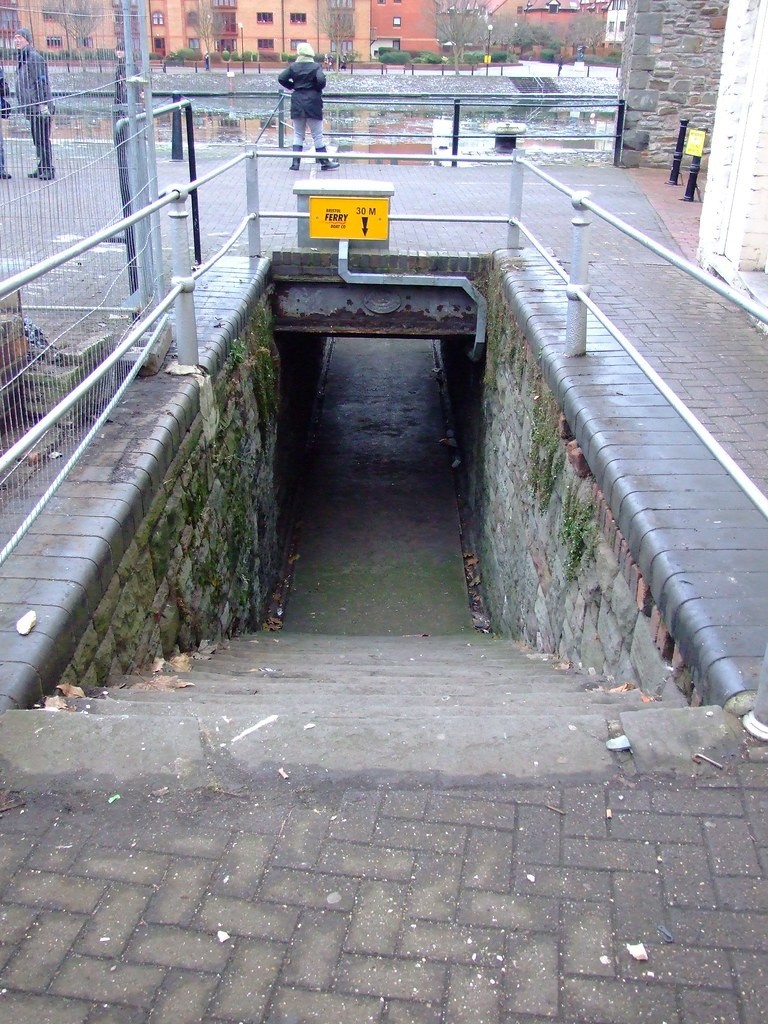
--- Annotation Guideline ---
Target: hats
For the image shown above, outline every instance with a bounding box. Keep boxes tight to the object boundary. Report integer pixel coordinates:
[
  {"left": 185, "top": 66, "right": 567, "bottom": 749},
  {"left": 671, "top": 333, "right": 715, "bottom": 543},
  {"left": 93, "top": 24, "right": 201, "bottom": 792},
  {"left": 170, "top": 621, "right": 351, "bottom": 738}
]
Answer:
[
  {"left": 15, "top": 28, "right": 32, "bottom": 44},
  {"left": 297, "top": 43, "right": 315, "bottom": 57}
]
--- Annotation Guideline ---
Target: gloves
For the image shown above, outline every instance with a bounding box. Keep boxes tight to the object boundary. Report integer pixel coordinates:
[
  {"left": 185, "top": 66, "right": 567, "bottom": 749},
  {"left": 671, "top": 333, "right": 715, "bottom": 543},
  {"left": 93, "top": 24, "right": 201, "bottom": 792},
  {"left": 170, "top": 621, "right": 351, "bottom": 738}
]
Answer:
[{"left": 41, "top": 105, "right": 50, "bottom": 119}]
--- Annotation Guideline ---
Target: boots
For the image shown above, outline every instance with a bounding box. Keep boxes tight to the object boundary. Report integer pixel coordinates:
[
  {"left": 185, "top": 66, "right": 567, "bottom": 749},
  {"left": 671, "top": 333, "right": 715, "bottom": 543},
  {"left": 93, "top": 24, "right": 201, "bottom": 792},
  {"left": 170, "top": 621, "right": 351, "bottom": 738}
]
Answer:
[
  {"left": 289, "top": 145, "right": 302, "bottom": 170},
  {"left": 316, "top": 147, "right": 340, "bottom": 169}
]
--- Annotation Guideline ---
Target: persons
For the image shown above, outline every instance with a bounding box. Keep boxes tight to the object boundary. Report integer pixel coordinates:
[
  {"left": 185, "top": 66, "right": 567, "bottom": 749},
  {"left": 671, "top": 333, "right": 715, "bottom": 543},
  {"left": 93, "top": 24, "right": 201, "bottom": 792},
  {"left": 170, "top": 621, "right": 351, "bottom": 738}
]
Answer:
[
  {"left": 203, "top": 51, "right": 209, "bottom": 70},
  {"left": 0, "top": 65, "right": 13, "bottom": 179},
  {"left": 113, "top": 40, "right": 140, "bottom": 118},
  {"left": 278, "top": 42, "right": 340, "bottom": 170},
  {"left": 328, "top": 55, "right": 335, "bottom": 71},
  {"left": 557, "top": 55, "right": 564, "bottom": 76},
  {"left": 341, "top": 56, "right": 347, "bottom": 70},
  {"left": 12, "top": 28, "right": 54, "bottom": 181},
  {"left": 324, "top": 54, "right": 329, "bottom": 71}
]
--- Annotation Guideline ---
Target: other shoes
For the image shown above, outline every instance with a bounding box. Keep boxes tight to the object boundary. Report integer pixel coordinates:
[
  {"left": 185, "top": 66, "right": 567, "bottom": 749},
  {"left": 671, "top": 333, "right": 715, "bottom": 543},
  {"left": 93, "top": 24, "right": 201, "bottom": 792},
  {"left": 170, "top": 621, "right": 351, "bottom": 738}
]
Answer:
[
  {"left": 0, "top": 173, "right": 11, "bottom": 179},
  {"left": 40, "top": 169, "right": 54, "bottom": 179},
  {"left": 28, "top": 171, "right": 42, "bottom": 178}
]
